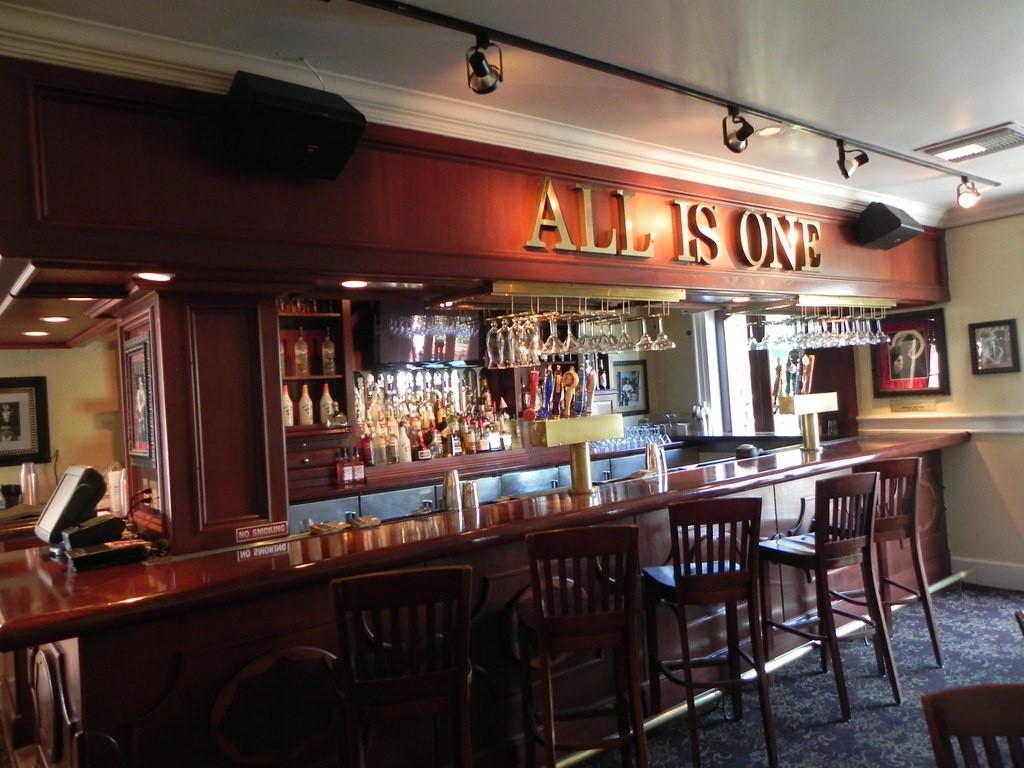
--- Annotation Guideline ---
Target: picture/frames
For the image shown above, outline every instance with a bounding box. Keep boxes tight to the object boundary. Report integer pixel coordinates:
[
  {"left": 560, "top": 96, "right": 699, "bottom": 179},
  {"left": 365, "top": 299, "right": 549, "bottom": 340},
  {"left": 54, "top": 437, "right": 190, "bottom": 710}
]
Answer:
[
  {"left": 0, "top": 374, "right": 54, "bottom": 467},
  {"left": 968, "top": 319, "right": 1021, "bottom": 375},
  {"left": 611, "top": 359, "right": 651, "bottom": 417},
  {"left": 122, "top": 330, "right": 158, "bottom": 471},
  {"left": 867, "top": 308, "right": 950, "bottom": 398}
]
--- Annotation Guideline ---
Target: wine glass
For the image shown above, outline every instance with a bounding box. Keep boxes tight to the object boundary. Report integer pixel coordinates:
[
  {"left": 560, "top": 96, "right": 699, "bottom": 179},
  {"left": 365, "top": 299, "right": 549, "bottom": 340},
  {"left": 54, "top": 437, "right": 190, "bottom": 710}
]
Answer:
[
  {"left": 486, "top": 312, "right": 677, "bottom": 371},
  {"left": 590, "top": 412, "right": 680, "bottom": 455},
  {"left": 744, "top": 315, "right": 892, "bottom": 352}
]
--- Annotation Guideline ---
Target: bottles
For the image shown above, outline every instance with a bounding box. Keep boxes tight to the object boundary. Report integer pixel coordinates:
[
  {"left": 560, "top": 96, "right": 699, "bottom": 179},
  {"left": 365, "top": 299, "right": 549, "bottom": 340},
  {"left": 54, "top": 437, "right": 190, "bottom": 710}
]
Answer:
[
  {"left": 336, "top": 447, "right": 353, "bottom": 486},
  {"left": 295, "top": 326, "right": 310, "bottom": 377},
  {"left": 325, "top": 400, "right": 347, "bottom": 429},
  {"left": 283, "top": 384, "right": 294, "bottom": 427},
  {"left": 319, "top": 383, "right": 334, "bottom": 424},
  {"left": 298, "top": 384, "right": 313, "bottom": 425},
  {"left": 690, "top": 400, "right": 711, "bottom": 435},
  {"left": 353, "top": 374, "right": 522, "bottom": 484},
  {"left": 322, "top": 326, "right": 336, "bottom": 376}
]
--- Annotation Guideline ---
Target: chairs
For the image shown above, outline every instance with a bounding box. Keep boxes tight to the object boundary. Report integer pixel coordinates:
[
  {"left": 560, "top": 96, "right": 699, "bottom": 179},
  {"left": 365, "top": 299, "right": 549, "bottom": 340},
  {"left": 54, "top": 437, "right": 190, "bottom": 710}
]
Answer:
[{"left": 919, "top": 681, "right": 1024, "bottom": 768}]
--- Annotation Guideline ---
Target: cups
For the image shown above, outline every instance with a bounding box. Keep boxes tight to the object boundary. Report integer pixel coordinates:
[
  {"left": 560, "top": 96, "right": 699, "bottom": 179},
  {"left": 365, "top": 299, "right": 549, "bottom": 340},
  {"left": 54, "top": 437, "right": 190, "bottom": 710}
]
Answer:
[
  {"left": 277, "top": 295, "right": 339, "bottom": 313},
  {"left": 441, "top": 470, "right": 479, "bottom": 511},
  {"left": 645, "top": 443, "right": 667, "bottom": 472},
  {"left": 20, "top": 462, "right": 38, "bottom": 505},
  {"left": 827, "top": 420, "right": 838, "bottom": 435},
  {"left": 445, "top": 507, "right": 480, "bottom": 534},
  {"left": 652, "top": 475, "right": 668, "bottom": 494}
]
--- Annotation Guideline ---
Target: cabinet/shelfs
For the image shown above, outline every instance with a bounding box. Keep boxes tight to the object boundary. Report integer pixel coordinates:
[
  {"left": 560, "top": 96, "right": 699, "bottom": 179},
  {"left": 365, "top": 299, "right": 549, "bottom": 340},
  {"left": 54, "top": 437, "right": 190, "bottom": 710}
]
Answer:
[
  {"left": 273, "top": 292, "right": 366, "bottom": 501},
  {"left": 511, "top": 310, "right": 585, "bottom": 420}
]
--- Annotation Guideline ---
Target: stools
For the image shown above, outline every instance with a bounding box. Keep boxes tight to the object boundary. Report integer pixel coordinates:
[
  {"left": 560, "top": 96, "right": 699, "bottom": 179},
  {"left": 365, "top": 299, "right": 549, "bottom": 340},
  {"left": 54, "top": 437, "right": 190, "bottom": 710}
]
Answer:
[{"left": 333, "top": 458, "right": 944, "bottom": 768}]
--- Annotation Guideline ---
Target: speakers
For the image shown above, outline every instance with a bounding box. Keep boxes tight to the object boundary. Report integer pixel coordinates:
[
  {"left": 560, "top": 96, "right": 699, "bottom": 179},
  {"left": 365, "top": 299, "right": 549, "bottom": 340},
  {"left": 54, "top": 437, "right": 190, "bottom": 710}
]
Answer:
[
  {"left": 212, "top": 71, "right": 367, "bottom": 181},
  {"left": 853, "top": 201, "right": 925, "bottom": 251}
]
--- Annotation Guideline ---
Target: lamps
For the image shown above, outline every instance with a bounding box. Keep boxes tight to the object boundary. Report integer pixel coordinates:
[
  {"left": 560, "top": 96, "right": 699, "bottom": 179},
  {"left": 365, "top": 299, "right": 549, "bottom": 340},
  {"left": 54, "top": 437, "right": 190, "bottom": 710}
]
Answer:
[
  {"left": 956, "top": 177, "right": 982, "bottom": 208},
  {"left": 836, "top": 140, "right": 869, "bottom": 178},
  {"left": 466, "top": 34, "right": 503, "bottom": 93},
  {"left": 722, "top": 107, "right": 755, "bottom": 152}
]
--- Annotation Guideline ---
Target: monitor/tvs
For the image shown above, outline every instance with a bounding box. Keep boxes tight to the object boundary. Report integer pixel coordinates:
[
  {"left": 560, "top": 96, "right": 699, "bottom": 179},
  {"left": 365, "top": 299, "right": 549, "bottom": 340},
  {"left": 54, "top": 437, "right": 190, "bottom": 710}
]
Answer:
[{"left": 34, "top": 465, "right": 107, "bottom": 545}]
